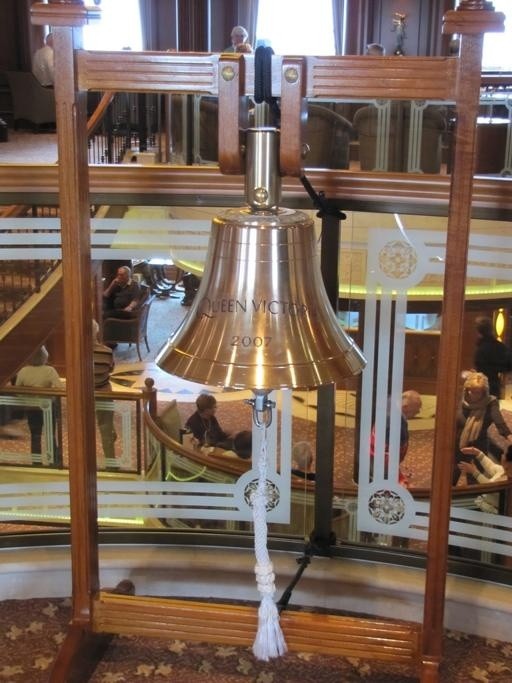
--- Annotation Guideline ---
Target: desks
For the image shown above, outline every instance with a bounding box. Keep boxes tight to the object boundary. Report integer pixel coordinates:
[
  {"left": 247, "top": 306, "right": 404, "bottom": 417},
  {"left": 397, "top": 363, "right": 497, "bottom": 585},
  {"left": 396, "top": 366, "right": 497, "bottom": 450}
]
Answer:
[{"left": 451, "top": 114, "right": 510, "bottom": 177}]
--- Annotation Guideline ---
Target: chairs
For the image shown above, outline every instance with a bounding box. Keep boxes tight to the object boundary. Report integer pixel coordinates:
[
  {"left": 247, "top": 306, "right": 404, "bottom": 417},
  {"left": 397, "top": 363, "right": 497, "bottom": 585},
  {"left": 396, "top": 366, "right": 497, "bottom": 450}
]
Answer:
[
  {"left": 100, "top": 292, "right": 158, "bottom": 363},
  {"left": 352, "top": 101, "right": 448, "bottom": 175},
  {"left": 270, "top": 103, "right": 356, "bottom": 170},
  {"left": 172, "top": 93, "right": 249, "bottom": 164}
]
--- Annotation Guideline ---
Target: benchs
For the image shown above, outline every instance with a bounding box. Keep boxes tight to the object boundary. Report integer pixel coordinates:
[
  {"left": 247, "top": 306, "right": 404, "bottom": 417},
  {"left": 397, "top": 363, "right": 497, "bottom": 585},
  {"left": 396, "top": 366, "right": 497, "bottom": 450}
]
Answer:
[{"left": 142, "top": 377, "right": 512, "bottom": 536}]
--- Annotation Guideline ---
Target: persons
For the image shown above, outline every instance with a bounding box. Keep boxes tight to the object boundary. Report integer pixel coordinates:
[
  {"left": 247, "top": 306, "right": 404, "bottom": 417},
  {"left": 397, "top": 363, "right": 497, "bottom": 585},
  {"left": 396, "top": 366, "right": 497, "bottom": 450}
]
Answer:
[
  {"left": 364, "top": 43, "right": 385, "bottom": 56},
  {"left": 472, "top": 314, "right": 512, "bottom": 401},
  {"left": 222, "top": 25, "right": 249, "bottom": 53},
  {"left": 232, "top": 42, "right": 255, "bottom": 54},
  {"left": 456, "top": 445, "right": 511, "bottom": 516},
  {"left": 29, "top": 31, "right": 54, "bottom": 91},
  {"left": 361, "top": 388, "right": 424, "bottom": 549},
  {"left": 14, "top": 344, "right": 66, "bottom": 466},
  {"left": 176, "top": 392, "right": 318, "bottom": 483},
  {"left": 101, "top": 264, "right": 144, "bottom": 351},
  {"left": 90, "top": 317, "right": 121, "bottom": 458},
  {"left": 449, "top": 370, "right": 512, "bottom": 485}
]
